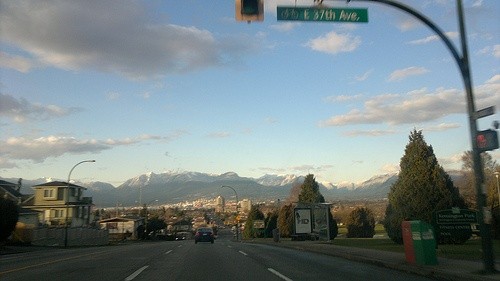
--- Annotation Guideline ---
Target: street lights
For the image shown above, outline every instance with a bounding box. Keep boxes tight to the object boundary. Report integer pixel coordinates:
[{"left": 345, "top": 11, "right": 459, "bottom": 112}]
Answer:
[
  {"left": 63, "top": 159, "right": 96, "bottom": 248},
  {"left": 222, "top": 186, "right": 238, "bottom": 241},
  {"left": 144, "top": 199, "right": 158, "bottom": 240}
]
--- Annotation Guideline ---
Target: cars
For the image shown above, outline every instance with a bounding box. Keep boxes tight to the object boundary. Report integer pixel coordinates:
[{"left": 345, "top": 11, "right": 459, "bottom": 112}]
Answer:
[{"left": 195, "top": 227, "right": 215, "bottom": 244}]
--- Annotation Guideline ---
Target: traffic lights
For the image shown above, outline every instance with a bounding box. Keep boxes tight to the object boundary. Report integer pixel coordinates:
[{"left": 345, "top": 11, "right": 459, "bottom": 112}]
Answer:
[
  {"left": 476, "top": 129, "right": 498, "bottom": 152},
  {"left": 235, "top": 0, "right": 264, "bottom": 22}
]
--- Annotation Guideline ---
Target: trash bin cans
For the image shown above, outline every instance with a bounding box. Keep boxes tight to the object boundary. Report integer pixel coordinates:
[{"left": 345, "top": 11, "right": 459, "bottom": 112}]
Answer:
[
  {"left": 272, "top": 228, "right": 279, "bottom": 241},
  {"left": 402, "top": 220, "right": 439, "bottom": 266}
]
